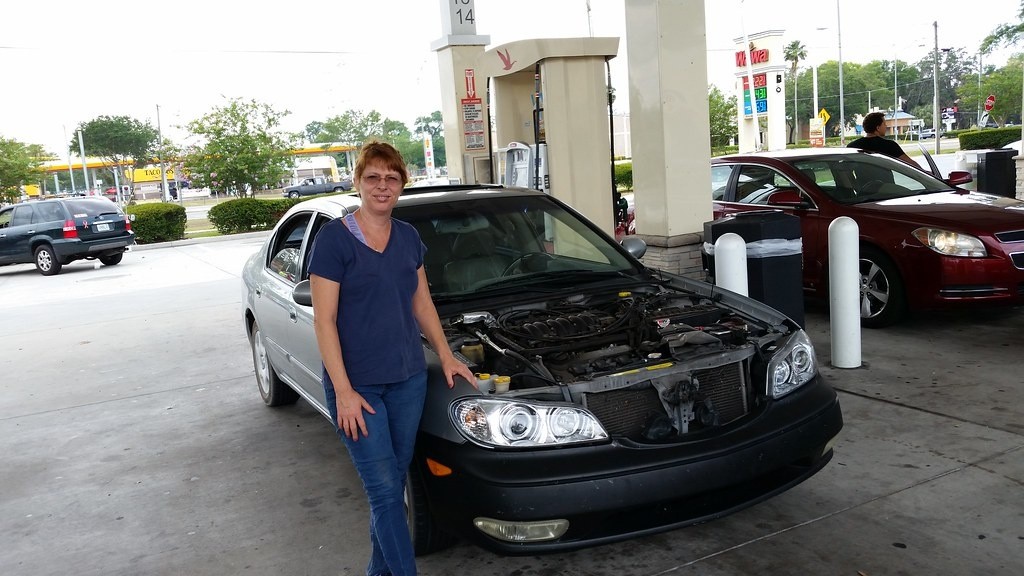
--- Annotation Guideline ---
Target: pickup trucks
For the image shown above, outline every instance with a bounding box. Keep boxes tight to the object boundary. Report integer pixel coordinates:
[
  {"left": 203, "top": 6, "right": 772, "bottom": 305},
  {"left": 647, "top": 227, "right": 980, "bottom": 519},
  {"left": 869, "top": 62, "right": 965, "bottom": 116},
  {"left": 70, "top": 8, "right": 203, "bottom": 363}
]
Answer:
[{"left": 281, "top": 175, "right": 352, "bottom": 200}]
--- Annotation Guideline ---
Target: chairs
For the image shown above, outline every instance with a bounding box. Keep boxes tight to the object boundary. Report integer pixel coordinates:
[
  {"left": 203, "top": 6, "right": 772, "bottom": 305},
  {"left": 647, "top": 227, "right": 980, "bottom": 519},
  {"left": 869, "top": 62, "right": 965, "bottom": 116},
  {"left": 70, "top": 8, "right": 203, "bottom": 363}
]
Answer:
[
  {"left": 444, "top": 231, "right": 509, "bottom": 292},
  {"left": 849, "top": 164, "right": 896, "bottom": 202}
]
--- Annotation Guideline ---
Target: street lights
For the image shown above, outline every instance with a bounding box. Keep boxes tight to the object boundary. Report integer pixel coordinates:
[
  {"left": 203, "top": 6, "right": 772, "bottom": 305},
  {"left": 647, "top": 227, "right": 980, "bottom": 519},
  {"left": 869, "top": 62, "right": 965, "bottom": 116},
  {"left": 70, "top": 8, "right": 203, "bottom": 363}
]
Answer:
[
  {"left": 916, "top": 21, "right": 941, "bottom": 156},
  {"left": 816, "top": 0, "right": 844, "bottom": 146}
]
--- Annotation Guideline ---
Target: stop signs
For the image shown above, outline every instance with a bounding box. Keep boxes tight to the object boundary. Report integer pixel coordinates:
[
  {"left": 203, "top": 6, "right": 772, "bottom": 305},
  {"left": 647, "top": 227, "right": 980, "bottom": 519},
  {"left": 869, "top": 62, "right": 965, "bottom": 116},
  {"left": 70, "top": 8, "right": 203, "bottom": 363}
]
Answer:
[{"left": 984, "top": 94, "right": 995, "bottom": 112}]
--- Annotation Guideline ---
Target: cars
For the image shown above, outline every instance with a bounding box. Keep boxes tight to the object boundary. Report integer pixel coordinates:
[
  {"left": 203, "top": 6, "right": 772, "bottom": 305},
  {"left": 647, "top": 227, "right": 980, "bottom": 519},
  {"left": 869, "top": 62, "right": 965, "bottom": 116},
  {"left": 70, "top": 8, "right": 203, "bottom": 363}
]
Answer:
[
  {"left": 80, "top": 184, "right": 132, "bottom": 203},
  {"left": 985, "top": 122, "right": 1015, "bottom": 129},
  {"left": 239, "top": 183, "right": 845, "bottom": 557},
  {"left": 0, "top": 195, "right": 136, "bottom": 276},
  {"left": 904, "top": 131, "right": 919, "bottom": 136},
  {"left": 923, "top": 129, "right": 943, "bottom": 139},
  {"left": 609, "top": 146, "right": 1024, "bottom": 329}
]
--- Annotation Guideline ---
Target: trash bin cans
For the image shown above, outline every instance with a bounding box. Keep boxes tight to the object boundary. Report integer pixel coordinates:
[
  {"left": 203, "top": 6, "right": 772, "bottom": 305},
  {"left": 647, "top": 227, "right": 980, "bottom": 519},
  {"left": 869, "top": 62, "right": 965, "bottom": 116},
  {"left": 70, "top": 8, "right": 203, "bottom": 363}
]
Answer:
[
  {"left": 701, "top": 210, "right": 804, "bottom": 331},
  {"left": 977, "top": 148, "right": 1019, "bottom": 199}
]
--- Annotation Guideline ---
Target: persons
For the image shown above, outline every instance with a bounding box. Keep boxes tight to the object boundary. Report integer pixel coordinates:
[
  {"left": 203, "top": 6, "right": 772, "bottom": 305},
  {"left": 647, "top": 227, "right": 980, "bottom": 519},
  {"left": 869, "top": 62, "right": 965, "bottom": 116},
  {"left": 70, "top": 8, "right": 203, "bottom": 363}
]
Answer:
[
  {"left": 841, "top": 113, "right": 933, "bottom": 193},
  {"left": 306, "top": 141, "right": 478, "bottom": 576}
]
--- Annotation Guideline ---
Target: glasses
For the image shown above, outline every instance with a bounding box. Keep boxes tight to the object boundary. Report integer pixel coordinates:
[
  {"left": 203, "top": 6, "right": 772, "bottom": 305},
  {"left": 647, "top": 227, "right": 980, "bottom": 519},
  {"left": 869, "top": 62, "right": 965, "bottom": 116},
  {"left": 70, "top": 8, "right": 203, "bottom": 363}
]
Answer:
[{"left": 360, "top": 176, "right": 402, "bottom": 184}]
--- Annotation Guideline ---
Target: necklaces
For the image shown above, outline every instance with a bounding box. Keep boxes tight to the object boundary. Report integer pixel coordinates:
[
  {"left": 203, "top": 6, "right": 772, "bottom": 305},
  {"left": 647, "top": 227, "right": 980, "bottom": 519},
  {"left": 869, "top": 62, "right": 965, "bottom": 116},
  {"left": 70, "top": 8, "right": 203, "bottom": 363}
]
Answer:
[{"left": 372, "top": 238, "right": 379, "bottom": 252}]
